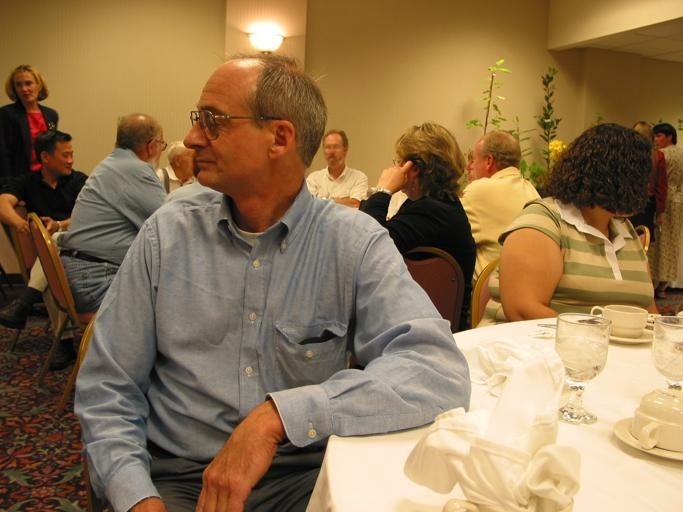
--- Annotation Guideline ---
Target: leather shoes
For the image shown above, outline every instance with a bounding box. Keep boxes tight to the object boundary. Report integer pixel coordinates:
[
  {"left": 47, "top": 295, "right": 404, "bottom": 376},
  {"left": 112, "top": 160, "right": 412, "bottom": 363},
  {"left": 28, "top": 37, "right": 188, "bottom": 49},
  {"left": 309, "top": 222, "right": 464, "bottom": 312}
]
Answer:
[
  {"left": 48, "top": 351, "right": 73, "bottom": 370},
  {"left": 0, "top": 299, "right": 27, "bottom": 329}
]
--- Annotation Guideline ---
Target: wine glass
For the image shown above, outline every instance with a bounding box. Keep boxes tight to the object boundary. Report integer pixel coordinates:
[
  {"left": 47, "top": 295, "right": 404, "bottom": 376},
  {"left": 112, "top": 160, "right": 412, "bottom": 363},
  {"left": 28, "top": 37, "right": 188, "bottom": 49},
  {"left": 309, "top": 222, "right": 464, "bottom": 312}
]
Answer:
[
  {"left": 554, "top": 312, "right": 611, "bottom": 424},
  {"left": 652, "top": 315, "right": 682, "bottom": 391}
]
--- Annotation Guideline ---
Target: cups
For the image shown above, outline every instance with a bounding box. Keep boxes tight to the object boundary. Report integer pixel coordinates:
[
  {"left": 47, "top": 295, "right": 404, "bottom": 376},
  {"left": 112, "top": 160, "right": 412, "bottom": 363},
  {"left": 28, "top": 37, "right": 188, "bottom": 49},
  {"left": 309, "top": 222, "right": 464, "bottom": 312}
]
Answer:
[
  {"left": 590, "top": 304, "right": 649, "bottom": 337},
  {"left": 630, "top": 391, "right": 682, "bottom": 452}
]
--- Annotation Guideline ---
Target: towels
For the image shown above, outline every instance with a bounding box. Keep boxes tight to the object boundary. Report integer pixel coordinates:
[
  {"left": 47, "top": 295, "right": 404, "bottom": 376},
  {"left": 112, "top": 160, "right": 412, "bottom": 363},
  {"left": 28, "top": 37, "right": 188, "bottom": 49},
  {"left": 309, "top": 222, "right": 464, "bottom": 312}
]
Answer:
[{"left": 406, "top": 342, "right": 577, "bottom": 512}]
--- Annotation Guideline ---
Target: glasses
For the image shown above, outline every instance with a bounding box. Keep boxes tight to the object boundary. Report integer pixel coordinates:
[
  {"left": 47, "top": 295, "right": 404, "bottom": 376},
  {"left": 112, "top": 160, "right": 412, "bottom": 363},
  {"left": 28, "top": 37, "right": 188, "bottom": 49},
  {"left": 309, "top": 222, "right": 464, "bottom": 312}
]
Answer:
[
  {"left": 157, "top": 140, "right": 167, "bottom": 150},
  {"left": 189, "top": 110, "right": 277, "bottom": 139}
]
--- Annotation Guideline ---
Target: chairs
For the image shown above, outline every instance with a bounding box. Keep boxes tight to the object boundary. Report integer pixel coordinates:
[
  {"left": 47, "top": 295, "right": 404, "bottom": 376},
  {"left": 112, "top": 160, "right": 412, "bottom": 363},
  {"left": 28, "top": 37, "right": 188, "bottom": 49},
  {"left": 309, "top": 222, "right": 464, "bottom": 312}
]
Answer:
[
  {"left": 633, "top": 224, "right": 649, "bottom": 254},
  {"left": 24, "top": 209, "right": 100, "bottom": 416},
  {"left": 7, "top": 205, "right": 58, "bottom": 355},
  {"left": 400, "top": 243, "right": 463, "bottom": 332},
  {"left": 471, "top": 257, "right": 504, "bottom": 329}
]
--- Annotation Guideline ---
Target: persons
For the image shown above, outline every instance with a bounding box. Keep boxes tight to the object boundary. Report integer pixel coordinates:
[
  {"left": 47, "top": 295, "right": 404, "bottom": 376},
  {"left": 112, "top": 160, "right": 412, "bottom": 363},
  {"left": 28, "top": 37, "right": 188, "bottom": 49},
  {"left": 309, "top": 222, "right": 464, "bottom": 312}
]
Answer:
[
  {"left": 306, "top": 129, "right": 369, "bottom": 208},
  {"left": 0, "top": 65, "right": 59, "bottom": 199},
  {"left": 458, "top": 129, "right": 543, "bottom": 300},
  {"left": 1, "top": 129, "right": 89, "bottom": 370},
  {"left": 156, "top": 139, "right": 202, "bottom": 195},
  {"left": 478, "top": 122, "right": 661, "bottom": 329},
  {"left": 630, "top": 120, "right": 669, "bottom": 253},
  {"left": 653, "top": 124, "right": 682, "bottom": 298},
  {"left": 358, "top": 123, "right": 477, "bottom": 330},
  {"left": 54, "top": 114, "right": 168, "bottom": 309},
  {"left": 71, "top": 47, "right": 472, "bottom": 512}
]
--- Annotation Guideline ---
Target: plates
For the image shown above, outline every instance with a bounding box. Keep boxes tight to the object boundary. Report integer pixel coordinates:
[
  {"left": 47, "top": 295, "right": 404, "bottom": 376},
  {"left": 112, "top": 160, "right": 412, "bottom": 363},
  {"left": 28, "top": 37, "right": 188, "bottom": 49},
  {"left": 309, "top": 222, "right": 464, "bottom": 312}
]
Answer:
[
  {"left": 608, "top": 331, "right": 652, "bottom": 345},
  {"left": 612, "top": 418, "right": 683, "bottom": 461}
]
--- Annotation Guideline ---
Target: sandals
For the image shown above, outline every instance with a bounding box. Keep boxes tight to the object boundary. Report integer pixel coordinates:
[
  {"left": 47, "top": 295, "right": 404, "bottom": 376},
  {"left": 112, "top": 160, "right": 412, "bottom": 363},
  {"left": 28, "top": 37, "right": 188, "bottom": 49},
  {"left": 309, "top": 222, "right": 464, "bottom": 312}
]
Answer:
[{"left": 654, "top": 289, "right": 665, "bottom": 298}]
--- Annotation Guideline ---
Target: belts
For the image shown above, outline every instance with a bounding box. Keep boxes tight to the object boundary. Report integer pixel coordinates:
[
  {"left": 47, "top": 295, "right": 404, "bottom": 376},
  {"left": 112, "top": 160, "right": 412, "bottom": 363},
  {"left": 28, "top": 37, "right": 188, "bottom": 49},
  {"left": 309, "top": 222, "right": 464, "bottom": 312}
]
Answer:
[{"left": 58, "top": 249, "right": 105, "bottom": 262}]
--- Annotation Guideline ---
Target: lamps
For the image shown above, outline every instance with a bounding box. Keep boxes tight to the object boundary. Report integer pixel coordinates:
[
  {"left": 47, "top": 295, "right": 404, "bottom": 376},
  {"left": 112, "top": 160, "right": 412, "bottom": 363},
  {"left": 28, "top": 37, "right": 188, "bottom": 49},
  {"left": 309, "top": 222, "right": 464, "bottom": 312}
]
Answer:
[{"left": 247, "top": 23, "right": 287, "bottom": 55}]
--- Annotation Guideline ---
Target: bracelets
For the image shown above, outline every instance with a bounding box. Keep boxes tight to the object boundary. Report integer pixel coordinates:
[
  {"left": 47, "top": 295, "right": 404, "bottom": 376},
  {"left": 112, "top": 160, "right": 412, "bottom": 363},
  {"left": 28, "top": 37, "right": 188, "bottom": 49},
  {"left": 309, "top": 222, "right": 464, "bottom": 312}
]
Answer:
[{"left": 371, "top": 186, "right": 392, "bottom": 194}]
